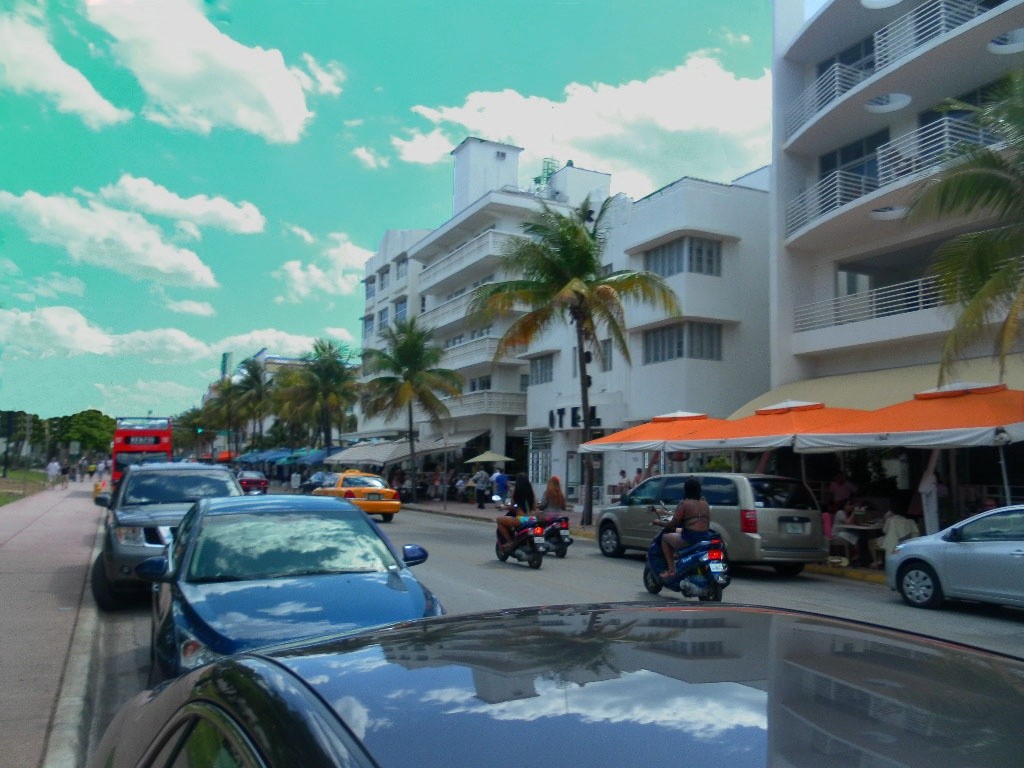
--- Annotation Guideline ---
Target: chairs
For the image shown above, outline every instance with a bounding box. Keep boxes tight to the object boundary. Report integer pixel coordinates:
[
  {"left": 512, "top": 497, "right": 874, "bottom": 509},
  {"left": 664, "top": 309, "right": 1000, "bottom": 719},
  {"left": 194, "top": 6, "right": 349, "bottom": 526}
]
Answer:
[{"left": 822, "top": 513, "right": 850, "bottom": 561}]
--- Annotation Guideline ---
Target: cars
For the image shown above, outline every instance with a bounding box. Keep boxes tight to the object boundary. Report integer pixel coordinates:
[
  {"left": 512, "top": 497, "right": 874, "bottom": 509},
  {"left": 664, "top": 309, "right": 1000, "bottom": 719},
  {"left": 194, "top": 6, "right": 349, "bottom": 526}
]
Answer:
[
  {"left": 84, "top": 599, "right": 1024, "bottom": 768},
  {"left": 884, "top": 504, "right": 1024, "bottom": 610},
  {"left": 310, "top": 469, "right": 402, "bottom": 522},
  {"left": 134, "top": 494, "right": 447, "bottom": 681},
  {"left": 297, "top": 470, "right": 336, "bottom": 494},
  {"left": 237, "top": 470, "right": 269, "bottom": 494}
]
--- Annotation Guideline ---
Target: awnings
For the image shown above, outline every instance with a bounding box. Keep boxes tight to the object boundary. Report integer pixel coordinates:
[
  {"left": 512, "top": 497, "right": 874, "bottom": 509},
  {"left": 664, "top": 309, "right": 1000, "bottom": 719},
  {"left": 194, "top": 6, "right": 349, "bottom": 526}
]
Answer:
[{"left": 438, "top": 428, "right": 492, "bottom": 448}]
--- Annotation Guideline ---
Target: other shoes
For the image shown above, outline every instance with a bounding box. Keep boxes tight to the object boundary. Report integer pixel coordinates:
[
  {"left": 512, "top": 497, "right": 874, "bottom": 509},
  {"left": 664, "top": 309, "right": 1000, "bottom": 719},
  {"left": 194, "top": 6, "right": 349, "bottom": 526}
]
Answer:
[
  {"left": 660, "top": 570, "right": 675, "bottom": 577},
  {"left": 502, "top": 541, "right": 514, "bottom": 548}
]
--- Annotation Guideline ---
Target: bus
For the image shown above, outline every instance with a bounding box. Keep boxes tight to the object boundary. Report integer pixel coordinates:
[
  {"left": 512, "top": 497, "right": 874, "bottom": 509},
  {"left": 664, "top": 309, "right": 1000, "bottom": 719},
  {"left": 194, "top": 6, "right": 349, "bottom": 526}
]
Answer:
[{"left": 109, "top": 416, "right": 175, "bottom": 491}]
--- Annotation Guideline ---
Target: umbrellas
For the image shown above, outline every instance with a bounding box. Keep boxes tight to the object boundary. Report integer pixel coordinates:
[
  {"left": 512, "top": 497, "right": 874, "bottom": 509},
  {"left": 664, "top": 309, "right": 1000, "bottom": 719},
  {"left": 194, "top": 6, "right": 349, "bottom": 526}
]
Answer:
[
  {"left": 234, "top": 434, "right": 456, "bottom": 476},
  {"left": 578, "top": 381, "right": 1024, "bottom": 509},
  {"left": 464, "top": 451, "right": 515, "bottom": 463}
]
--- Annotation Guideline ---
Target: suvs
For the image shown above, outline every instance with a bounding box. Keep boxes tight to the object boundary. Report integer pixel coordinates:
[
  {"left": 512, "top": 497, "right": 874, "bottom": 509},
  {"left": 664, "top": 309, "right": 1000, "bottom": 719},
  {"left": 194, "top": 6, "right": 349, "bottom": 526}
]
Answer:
[
  {"left": 91, "top": 461, "right": 263, "bottom": 611},
  {"left": 595, "top": 473, "right": 832, "bottom": 579}
]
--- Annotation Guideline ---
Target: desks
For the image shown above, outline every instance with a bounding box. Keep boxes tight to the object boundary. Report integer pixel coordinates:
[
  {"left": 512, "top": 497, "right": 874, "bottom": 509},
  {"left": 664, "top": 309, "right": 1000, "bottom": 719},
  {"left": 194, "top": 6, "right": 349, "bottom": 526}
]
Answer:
[{"left": 840, "top": 524, "right": 882, "bottom": 529}]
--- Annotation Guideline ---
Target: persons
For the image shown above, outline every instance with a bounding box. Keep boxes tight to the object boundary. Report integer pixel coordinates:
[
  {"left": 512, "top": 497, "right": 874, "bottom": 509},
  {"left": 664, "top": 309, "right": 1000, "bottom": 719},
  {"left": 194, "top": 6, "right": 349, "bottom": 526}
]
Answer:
[
  {"left": 47, "top": 454, "right": 112, "bottom": 490},
  {"left": 634, "top": 468, "right": 643, "bottom": 487},
  {"left": 618, "top": 470, "right": 630, "bottom": 494},
  {"left": 653, "top": 478, "right": 709, "bottom": 578},
  {"left": 496, "top": 473, "right": 537, "bottom": 548},
  {"left": 472, "top": 465, "right": 489, "bottom": 509},
  {"left": 488, "top": 468, "right": 510, "bottom": 509},
  {"left": 388, "top": 462, "right": 406, "bottom": 490},
  {"left": 447, "top": 466, "right": 469, "bottom": 502},
  {"left": 824, "top": 473, "right": 906, "bottom": 569},
  {"left": 432, "top": 464, "right": 441, "bottom": 498},
  {"left": 536, "top": 476, "right": 566, "bottom": 522}
]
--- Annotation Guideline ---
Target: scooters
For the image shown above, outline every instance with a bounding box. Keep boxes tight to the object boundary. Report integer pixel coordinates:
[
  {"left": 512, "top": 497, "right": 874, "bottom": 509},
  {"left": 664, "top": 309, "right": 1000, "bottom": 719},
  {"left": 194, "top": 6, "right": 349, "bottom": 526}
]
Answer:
[
  {"left": 642, "top": 500, "right": 732, "bottom": 601},
  {"left": 491, "top": 495, "right": 547, "bottom": 570},
  {"left": 535, "top": 502, "right": 574, "bottom": 557}
]
